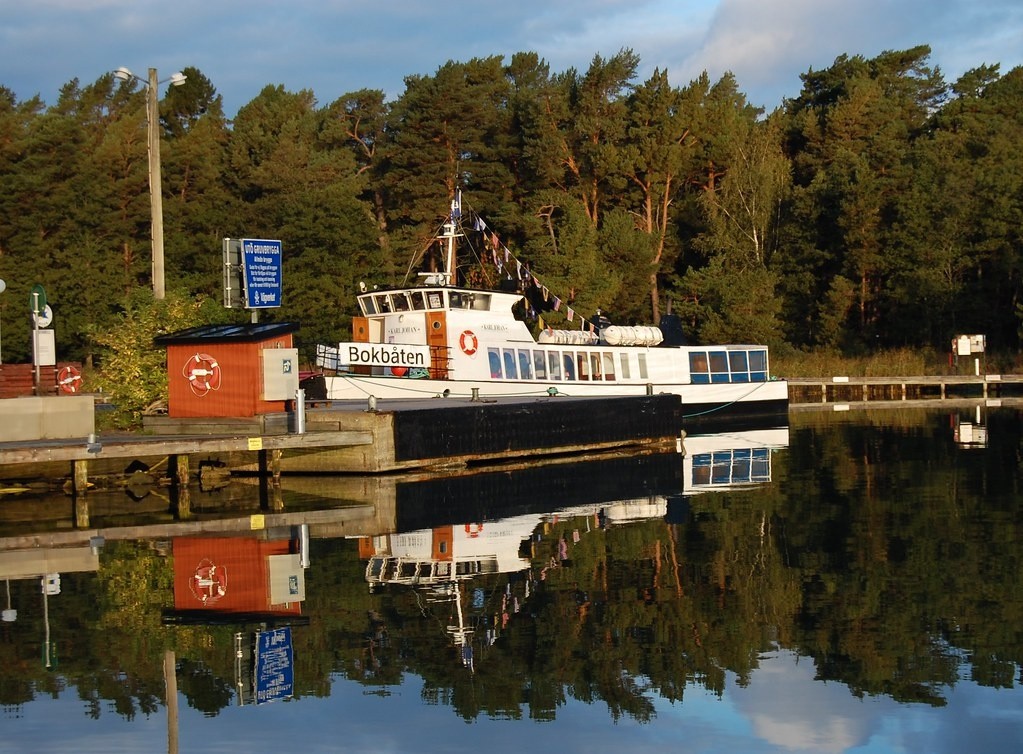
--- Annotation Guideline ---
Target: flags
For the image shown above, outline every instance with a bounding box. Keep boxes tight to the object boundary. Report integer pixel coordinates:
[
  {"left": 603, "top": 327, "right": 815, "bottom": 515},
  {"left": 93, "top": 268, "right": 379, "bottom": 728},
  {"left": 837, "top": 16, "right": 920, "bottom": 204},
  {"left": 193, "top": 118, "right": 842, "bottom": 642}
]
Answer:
[
  {"left": 477, "top": 507, "right": 600, "bottom": 650},
  {"left": 450, "top": 205, "right": 596, "bottom": 339}
]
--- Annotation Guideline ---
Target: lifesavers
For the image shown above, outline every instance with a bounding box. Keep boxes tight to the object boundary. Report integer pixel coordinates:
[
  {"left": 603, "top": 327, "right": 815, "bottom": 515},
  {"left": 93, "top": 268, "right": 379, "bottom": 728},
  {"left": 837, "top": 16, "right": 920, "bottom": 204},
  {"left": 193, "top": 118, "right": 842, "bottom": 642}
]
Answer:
[
  {"left": 186, "top": 353, "right": 219, "bottom": 390},
  {"left": 465, "top": 522, "right": 483, "bottom": 537},
  {"left": 460, "top": 330, "right": 478, "bottom": 355},
  {"left": 57, "top": 366, "right": 82, "bottom": 393},
  {"left": 194, "top": 567, "right": 225, "bottom": 602}
]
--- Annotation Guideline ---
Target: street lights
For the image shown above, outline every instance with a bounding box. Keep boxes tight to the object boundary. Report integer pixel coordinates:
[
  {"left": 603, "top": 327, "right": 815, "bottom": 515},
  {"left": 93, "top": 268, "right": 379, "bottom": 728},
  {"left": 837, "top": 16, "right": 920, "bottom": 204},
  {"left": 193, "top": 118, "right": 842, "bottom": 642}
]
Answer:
[{"left": 115, "top": 64, "right": 187, "bottom": 299}]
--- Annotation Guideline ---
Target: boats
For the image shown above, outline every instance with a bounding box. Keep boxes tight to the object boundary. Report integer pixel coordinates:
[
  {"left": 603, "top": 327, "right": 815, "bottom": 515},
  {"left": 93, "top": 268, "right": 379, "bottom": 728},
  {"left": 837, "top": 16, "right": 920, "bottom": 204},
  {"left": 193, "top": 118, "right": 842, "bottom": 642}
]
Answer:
[
  {"left": 313, "top": 157, "right": 791, "bottom": 419},
  {"left": 358, "top": 422, "right": 791, "bottom": 677}
]
